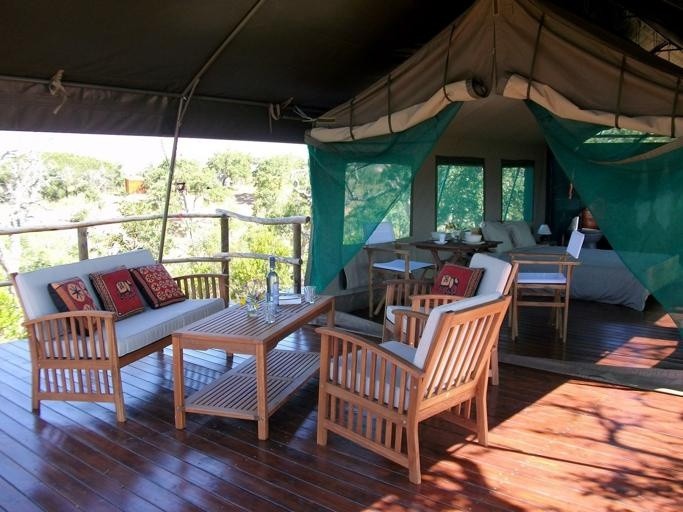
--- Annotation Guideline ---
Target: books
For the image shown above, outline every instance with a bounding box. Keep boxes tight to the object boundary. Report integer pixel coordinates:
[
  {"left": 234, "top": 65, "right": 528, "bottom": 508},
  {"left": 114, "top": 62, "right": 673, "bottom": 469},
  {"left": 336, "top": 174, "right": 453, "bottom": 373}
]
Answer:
[{"left": 270, "top": 293, "right": 302, "bottom": 305}]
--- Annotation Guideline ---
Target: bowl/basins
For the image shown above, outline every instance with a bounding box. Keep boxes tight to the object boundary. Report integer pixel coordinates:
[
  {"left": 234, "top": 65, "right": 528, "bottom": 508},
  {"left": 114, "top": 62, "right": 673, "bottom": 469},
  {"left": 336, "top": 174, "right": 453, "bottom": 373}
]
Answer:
[
  {"left": 431, "top": 232, "right": 444, "bottom": 238},
  {"left": 465, "top": 235, "right": 482, "bottom": 242}
]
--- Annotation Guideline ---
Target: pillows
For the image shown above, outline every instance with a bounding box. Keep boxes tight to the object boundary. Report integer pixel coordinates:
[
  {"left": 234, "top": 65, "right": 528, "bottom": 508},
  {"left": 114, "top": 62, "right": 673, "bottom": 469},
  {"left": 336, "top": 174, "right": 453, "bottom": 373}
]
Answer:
[
  {"left": 480, "top": 221, "right": 514, "bottom": 253},
  {"left": 88, "top": 266, "right": 146, "bottom": 322},
  {"left": 129, "top": 265, "right": 187, "bottom": 308},
  {"left": 47, "top": 276, "right": 103, "bottom": 335},
  {"left": 505, "top": 222, "right": 537, "bottom": 248},
  {"left": 422, "top": 263, "right": 484, "bottom": 305}
]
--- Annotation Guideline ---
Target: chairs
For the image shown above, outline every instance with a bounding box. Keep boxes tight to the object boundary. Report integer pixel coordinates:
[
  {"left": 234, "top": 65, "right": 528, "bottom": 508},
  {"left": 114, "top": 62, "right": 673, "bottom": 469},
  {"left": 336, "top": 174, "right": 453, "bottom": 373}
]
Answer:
[
  {"left": 362, "top": 240, "right": 437, "bottom": 318},
  {"left": 382, "top": 252, "right": 520, "bottom": 391},
  {"left": 508, "top": 231, "right": 586, "bottom": 344},
  {"left": 314, "top": 295, "right": 512, "bottom": 484}
]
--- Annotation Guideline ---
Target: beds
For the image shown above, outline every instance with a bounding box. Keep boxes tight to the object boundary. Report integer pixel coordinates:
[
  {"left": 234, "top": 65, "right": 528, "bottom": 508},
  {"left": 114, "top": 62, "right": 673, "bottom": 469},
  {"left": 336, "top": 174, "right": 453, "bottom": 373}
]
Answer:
[{"left": 477, "top": 222, "right": 681, "bottom": 311}]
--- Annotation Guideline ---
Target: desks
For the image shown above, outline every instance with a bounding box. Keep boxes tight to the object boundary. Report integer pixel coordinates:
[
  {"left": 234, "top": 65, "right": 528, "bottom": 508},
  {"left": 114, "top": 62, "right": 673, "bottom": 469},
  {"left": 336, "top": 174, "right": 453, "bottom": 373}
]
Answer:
[
  {"left": 169, "top": 293, "right": 335, "bottom": 440},
  {"left": 411, "top": 240, "right": 504, "bottom": 274}
]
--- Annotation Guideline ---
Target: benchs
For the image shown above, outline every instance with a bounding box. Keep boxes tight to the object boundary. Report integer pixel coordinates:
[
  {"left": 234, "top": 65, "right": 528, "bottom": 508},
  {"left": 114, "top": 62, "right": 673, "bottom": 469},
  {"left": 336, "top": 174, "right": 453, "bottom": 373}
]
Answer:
[{"left": 11, "top": 262, "right": 230, "bottom": 423}]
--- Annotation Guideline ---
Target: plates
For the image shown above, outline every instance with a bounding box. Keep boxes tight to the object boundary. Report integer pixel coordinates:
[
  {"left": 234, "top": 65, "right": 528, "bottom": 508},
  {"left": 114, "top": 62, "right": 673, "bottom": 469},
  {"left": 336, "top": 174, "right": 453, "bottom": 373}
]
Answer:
[
  {"left": 462, "top": 241, "right": 485, "bottom": 245},
  {"left": 434, "top": 241, "right": 448, "bottom": 245}
]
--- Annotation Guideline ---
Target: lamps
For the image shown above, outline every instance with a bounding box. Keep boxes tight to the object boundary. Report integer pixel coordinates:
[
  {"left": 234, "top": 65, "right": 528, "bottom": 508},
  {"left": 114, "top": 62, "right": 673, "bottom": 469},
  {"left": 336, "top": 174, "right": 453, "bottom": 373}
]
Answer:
[{"left": 538, "top": 224, "right": 552, "bottom": 245}]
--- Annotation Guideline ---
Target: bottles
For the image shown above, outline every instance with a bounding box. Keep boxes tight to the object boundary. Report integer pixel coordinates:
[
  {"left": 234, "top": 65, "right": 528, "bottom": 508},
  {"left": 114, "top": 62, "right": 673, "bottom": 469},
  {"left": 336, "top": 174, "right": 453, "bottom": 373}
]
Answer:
[{"left": 266, "top": 256, "right": 279, "bottom": 306}]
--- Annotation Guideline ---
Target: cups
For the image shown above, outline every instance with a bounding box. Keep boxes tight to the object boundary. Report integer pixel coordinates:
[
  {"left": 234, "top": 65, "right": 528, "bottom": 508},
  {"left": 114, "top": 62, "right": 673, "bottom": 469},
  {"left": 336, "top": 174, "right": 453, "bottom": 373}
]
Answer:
[
  {"left": 268, "top": 304, "right": 275, "bottom": 323},
  {"left": 439, "top": 234, "right": 446, "bottom": 241},
  {"left": 304, "top": 287, "right": 310, "bottom": 302},
  {"left": 265, "top": 304, "right": 269, "bottom": 321},
  {"left": 309, "top": 286, "right": 315, "bottom": 304}
]
